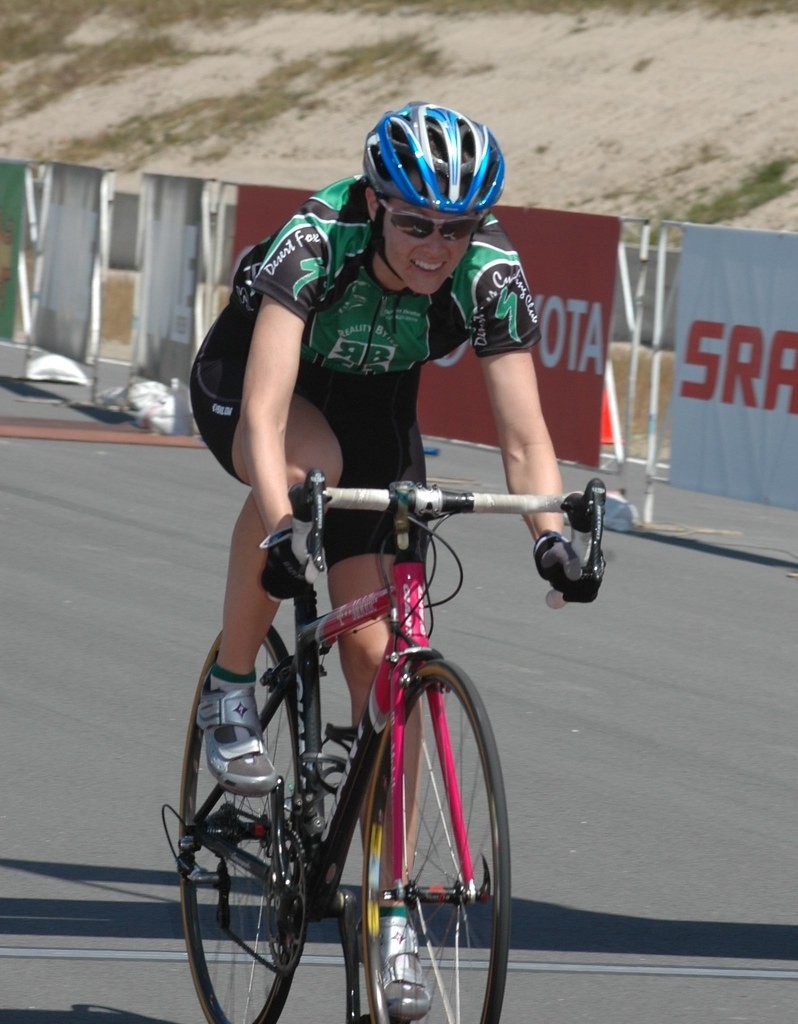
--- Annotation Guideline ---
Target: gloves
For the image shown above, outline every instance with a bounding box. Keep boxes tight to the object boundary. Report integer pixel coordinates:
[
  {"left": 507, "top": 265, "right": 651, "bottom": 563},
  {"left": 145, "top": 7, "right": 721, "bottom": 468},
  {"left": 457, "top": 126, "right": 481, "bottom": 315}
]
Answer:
[
  {"left": 533, "top": 530, "right": 607, "bottom": 605},
  {"left": 256, "top": 527, "right": 314, "bottom": 606}
]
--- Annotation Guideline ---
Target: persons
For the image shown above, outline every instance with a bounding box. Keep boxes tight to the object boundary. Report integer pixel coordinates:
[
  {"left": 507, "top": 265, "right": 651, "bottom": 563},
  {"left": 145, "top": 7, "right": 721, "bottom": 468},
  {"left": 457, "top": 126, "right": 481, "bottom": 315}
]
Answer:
[{"left": 189, "top": 99, "right": 603, "bottom": 1021}]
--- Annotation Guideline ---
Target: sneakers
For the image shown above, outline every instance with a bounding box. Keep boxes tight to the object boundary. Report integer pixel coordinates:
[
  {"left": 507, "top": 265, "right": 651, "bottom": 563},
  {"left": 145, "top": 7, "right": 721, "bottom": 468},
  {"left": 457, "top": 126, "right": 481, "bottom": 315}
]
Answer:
[
  {"left": 198, "top": 677, "right": 282, "bottom": 797},
  {"left": 370, "top": 911, "right": 433, "bottom": 1020}
]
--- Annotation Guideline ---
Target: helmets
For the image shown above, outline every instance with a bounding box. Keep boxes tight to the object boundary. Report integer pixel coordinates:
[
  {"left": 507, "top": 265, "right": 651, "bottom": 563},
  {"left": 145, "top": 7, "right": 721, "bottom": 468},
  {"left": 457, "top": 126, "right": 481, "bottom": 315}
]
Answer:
[{"left": 365, "top": 102, "right": 504, "bottom": 217}]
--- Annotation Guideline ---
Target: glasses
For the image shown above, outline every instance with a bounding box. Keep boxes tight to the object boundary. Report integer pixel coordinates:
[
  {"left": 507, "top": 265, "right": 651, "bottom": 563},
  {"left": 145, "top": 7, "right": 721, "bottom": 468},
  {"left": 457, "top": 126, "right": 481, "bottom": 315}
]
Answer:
[{"left": 371, "top": 198, "right": 486, "bottom": 243}]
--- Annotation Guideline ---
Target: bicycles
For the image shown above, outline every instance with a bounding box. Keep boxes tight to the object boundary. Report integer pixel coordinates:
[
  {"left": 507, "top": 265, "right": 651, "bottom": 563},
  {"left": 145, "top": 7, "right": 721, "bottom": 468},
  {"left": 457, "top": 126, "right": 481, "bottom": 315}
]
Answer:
[{"left": 163, "top": 479, "right": 606, "bottom": 1021}]
